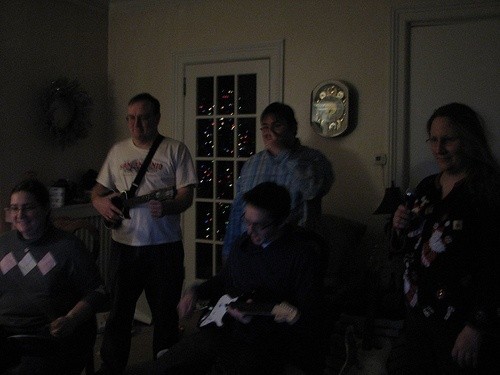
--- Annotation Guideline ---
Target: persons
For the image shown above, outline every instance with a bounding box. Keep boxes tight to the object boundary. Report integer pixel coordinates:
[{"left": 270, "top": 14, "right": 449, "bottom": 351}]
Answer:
[
  {"left": 387, "top": 102, "right": 500, "bottom": 375},
  {"left": 179, "top": 182, "right": 344, "bottom": 375},
  {"left": 92, "top": 93, "right": 198, "bottom": 375},
  {"left": 222, "top": 103, "right": 334, "bottom": 268},
  {"left": 0, "top": 180, "right": 110, "bottom": 375}
]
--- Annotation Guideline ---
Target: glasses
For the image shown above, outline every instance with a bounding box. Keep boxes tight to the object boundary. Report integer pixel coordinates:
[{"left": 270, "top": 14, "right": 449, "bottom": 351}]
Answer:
[
  {"left": 258, "top": 120, "right": 288, "bottom": 131},
  {"left": 125, "top": 113, "right": 157, "bottom": 123},
  {"left": 424, "top": 133, "right": 458, "bottom": 146},
  {"left": 239, "top": 210, "right": 276, "bottom": 232},
  {"left": 6, "top": 203, "right": 37, "bottom": 214}
]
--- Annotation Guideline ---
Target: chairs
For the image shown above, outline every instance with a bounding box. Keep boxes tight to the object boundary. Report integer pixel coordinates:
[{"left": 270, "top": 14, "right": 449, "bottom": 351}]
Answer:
[
  {"left": 195, "top": 216, "right": 368, "bottom": 375},
  {"left": 0, "top": 209, "right": 102, "bottom": 375}
]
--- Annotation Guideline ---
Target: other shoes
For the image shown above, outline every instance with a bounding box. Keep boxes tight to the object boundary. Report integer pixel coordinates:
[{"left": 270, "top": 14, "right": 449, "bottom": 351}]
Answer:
[{"left": 155, "top": 348, "right": 170, "bottom": 359}]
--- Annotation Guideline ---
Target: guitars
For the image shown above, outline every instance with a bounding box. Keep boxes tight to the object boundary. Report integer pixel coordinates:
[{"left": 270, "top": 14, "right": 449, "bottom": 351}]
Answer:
[
  {"left": 104, "top": 186, "right": 178, "bottom": 229},
  {"left": 196, "top": 293, "right": 301, "bottom": 330}
]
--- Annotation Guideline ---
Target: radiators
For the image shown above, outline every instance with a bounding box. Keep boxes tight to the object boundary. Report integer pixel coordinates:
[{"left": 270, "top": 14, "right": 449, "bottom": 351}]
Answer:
[{"left": 5, "top": 201, "right": 112, "bottom": 282}]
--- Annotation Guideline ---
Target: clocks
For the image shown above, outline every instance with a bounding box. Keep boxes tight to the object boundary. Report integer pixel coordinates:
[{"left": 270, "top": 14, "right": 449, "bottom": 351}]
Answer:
[{"left": 309, "top": 79, "right": 353, "bottom": 139}]
